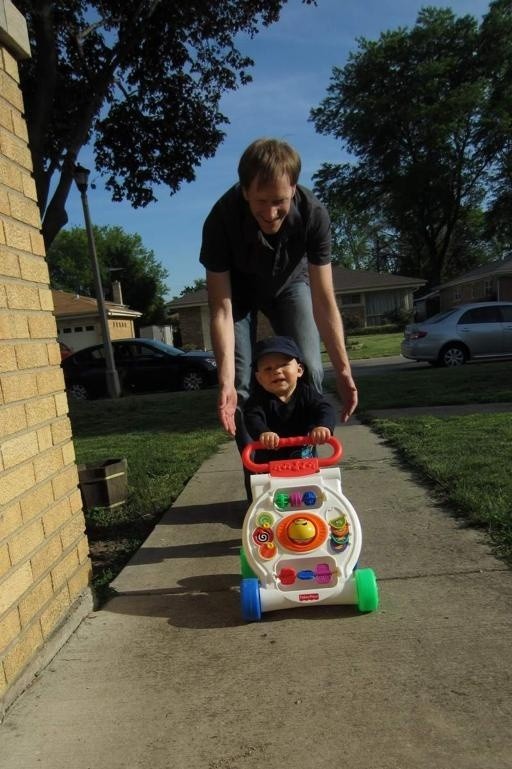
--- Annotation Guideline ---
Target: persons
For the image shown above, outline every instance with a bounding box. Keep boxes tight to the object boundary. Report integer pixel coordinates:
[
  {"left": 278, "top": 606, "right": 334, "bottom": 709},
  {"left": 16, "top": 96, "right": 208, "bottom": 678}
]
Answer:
[
  {"left": 200, "top": 139, "right": 362, "bottom": 508},
  {"left": 240, "top": 335, "right": 337, "bottom": 472}
]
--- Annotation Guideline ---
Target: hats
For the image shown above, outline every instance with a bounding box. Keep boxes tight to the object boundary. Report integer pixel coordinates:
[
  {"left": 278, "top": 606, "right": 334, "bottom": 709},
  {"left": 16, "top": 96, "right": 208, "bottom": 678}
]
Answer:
[{"left": 250, "top": 336, "right": 301, "bottom": 367}]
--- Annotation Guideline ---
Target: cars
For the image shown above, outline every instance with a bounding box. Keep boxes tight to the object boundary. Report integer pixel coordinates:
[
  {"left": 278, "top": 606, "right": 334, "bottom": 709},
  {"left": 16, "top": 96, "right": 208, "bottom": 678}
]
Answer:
[
  {"left": 61, "top": 338, "right": 220, "bottom": 402},
  {"left": 398, "top": 301, "right": 512, "bottom": 366}
]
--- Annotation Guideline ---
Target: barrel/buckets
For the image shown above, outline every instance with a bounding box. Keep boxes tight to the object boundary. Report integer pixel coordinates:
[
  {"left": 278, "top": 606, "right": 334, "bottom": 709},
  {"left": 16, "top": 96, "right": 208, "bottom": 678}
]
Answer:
[{"left": 77, "top": 457, "right": 129, "bottom": 507}]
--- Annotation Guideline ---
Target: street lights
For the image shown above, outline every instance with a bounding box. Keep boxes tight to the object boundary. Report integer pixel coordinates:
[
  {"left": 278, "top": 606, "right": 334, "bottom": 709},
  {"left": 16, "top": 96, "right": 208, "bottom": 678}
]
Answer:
[{"left": 70, "top": 162, "right": 123, "bottom": 403}]
